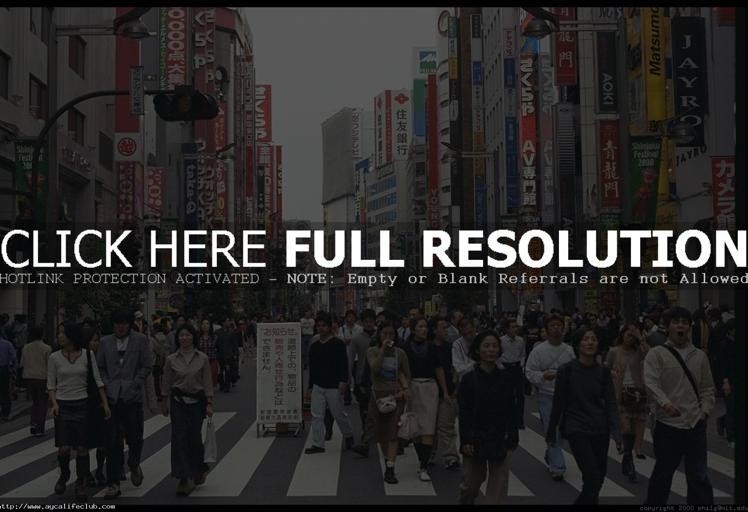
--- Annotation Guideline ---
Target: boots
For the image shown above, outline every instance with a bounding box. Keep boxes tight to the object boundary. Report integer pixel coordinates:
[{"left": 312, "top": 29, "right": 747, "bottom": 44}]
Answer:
[{"left": 384, "top": 465, "right": 399, "bottom": 484}]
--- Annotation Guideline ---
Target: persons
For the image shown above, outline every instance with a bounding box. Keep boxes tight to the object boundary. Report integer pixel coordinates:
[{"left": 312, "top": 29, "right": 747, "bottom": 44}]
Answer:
[{"left": 1, "top": 296, "right": 739, "bottom": 502}]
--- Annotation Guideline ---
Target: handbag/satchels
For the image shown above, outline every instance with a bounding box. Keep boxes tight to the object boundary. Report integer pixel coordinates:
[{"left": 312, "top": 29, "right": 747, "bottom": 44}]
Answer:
[
  {"left": 622, "top": 386, "right": 648, "bottom": 413},
  {"left": 375, "top": 395, "right": 396, "bottom": 413},
  {"left": 397, "top": 413, "right": 419, "bottom": 438},
  {"left": 204, "top": 416, "right": 218, "bottom": 463}
]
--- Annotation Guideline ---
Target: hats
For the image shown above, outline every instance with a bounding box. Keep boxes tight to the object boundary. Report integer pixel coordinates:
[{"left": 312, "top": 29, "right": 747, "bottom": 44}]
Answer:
[{"left": 134, "top": 311, "right": 143, "bottom": 319}]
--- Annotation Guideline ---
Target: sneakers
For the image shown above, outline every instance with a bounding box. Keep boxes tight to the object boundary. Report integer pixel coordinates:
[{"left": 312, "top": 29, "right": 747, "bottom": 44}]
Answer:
[
  {"left": 352, "top": 444, "right": 368, "bottom": 458},
  {"left": 304, "top": 445, "right": 325, "bottom": 455},
  {"left": 175, "top": 477, "right": 195, "bottom": 495},
  {"left": 55, "top": 468, "right": 143, "bottom": 497}
]
